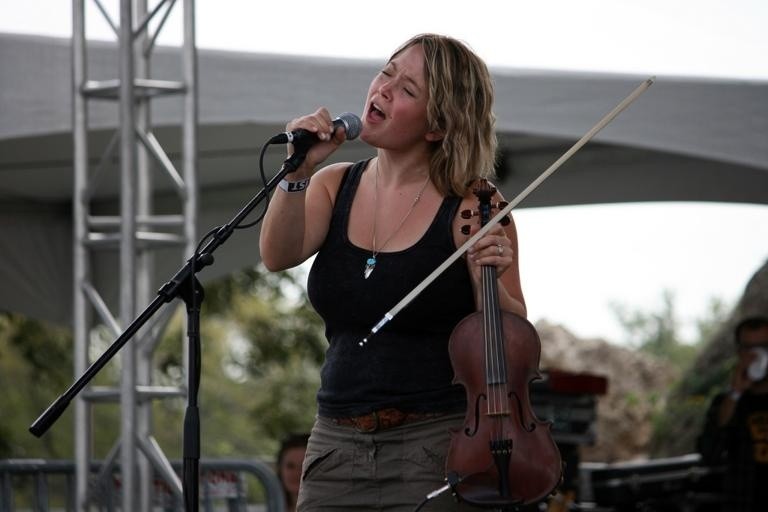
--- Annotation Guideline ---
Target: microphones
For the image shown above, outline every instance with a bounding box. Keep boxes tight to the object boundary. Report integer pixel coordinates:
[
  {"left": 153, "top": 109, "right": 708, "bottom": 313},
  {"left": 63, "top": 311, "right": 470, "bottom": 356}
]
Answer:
[{"left": 269, "top": 111, "right": 364, "bottom": 144}]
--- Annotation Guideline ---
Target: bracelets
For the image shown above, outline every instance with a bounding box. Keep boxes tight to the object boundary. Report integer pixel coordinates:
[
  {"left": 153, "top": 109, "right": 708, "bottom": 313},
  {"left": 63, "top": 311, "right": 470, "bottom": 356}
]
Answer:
[
  {"left": 728, "top": 392, "right": 743, "bottom": 402},
  {"left": 277, "top": 177, "right": 311, "bottom": 193}
]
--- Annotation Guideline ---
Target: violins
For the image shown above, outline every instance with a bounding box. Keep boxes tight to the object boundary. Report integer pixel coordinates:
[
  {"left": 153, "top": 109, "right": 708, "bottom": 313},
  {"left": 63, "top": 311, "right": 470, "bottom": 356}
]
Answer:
[{"left": 447, "top": 178, "right": 562, "bottom": 506}]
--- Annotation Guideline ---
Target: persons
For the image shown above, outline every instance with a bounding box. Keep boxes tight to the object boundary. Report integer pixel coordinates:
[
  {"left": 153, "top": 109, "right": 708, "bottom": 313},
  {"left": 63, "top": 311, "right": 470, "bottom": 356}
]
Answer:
[
  {"left": 698, "top": 316, "right": 768, "bottom": 511},
  {"left": 272, "top": 432, "right": 312, "bottom": 511},
  {"left": 257, "top": 31, "right": 529, "bottom": 510}
]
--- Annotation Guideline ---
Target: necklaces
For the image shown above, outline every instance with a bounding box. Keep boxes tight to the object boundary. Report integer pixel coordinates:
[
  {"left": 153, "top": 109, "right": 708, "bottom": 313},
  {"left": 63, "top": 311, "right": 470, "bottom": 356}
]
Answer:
[{"left": 363, "top": 155, "right": 431, "bottom": 279}]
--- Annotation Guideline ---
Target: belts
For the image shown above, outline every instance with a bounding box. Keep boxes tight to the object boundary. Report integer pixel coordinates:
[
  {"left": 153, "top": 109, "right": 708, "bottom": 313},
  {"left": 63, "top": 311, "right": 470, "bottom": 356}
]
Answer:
[{"left": 340, "top": 410, "right": 434, "bottom": 434}]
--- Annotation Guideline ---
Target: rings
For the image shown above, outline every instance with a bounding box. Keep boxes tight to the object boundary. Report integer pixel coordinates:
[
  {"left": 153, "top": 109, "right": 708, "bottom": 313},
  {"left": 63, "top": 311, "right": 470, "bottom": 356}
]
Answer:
[{"left": 498, "top": 244, "right": 503, "bottom": 257}]
